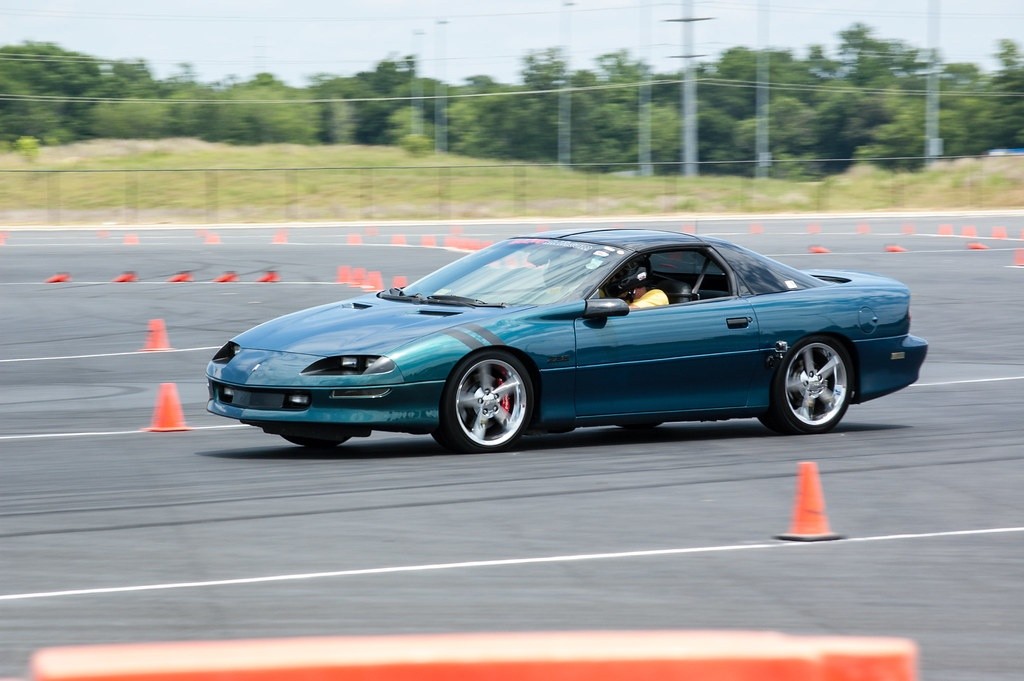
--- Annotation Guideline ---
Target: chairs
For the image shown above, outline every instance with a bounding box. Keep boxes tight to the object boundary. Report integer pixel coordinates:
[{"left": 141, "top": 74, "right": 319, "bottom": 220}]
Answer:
[{"left": 656, "top": 279, "right": 694, "bottom": 306}]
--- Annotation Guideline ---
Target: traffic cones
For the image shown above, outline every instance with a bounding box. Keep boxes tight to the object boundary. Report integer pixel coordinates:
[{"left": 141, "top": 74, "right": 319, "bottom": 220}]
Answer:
[
  {"left": 112, "top": 267, "right": 139, "bottom": 284},
  {"left": 856, "top": 221, "right": 871, "bottom": 233},
  {"left": 938, "top": 223, "right": 953, "bottom": 239},
  {"left": 750, "top": 221, "right": 762, "bottom": 237},
  {"left": 43, "top": 270, "right": 72, "bottom": 285},
  {"left": 363, "top": 272, "right": 381, "bottom": 293},
  {"left": 198, "top": 226, "right": 220, "bottom": 249},
  {"left": 146, "top": 382, "right": 195, "bottom": 432},
  {"left": 1013, "top": 248, "right": 1024, "bottom": 270},
  {"left": 275, "top": 226, "right": 289, "bottom": 243},
  {"left": 337, "top": 261, "right": 355, "bottom": 281},
  {"left": 347, "top": 266, "right": 367, "bottom": 287},
  {"left": 962, "top": 227, "right": 979, "bottom": 242},
  {"left": 256, "top": 268, "right": 279, "bottom": 284},
  {"left": 881, "top": 244, "right": 905, "bottom": 254},
  {"left": 807, "top": 245, "right": 831, "bottom": 256},
  {"left": 124, "top": 230, "right": 141, "bottom": 247},
  {"left": 775, "top": 462, "right": 851, "bottom": 545},
  {"left": 808, "top": 221, "right": 819, "bottom": 233},
  {"left": 169, "top": 270, "right": 196, "bottom": 284},
  {"left": 136, "top": 320, "right": 177, "bottom": 353},
  {"left": 901, "top": 222, "right": 918, "bottom": 235},
  {"left": 214, "top": 269, "right": 239, "bottom": 285},
  {"left": 389, "top": 273, "right": 404, "bottom": 296},
  {"left": 987, "top": 224, "right": 1024, "bottom": 241},
  {"left": 679, "top": 222, "right": 697, "bottom": 240},
  {"left": 346, "top": 228, "right": 497, "bottom": 259},
  {"left": 964, "top": 240, "right": 993, "bottom": 251}
]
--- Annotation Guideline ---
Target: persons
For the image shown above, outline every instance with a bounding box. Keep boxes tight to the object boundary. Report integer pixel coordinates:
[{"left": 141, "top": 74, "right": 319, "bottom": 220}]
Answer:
[{"left": 548, "top": 256, "right": 670, "bottom": 309}]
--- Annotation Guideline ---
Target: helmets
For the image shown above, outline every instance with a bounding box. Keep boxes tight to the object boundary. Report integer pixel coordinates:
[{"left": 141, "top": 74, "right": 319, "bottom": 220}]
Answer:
[{"left": 599, "top": 255, "right": 651, "bottom": 298}]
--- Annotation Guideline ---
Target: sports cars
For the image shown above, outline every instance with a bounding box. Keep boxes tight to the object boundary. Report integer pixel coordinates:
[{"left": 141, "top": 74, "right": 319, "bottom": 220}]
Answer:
[{"left": 202, "top": 227, "right": 930, "bottom": 457}]
